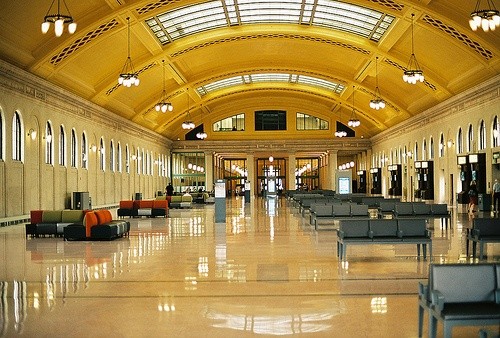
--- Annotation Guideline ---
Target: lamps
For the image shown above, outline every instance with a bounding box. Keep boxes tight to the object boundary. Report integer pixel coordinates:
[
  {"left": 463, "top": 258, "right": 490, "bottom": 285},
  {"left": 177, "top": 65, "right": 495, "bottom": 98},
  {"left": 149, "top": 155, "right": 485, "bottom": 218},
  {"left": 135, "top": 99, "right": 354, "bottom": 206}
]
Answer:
[
  {"left": 118, "top": 17, "right": 140, "bottom": 87},
  {"left": 155, "top": 60, "right": 173, "bottom": 112},
  {"left": 182, "top": 95, "right": 195, "bottom": 130},
  {"left": 348, "top": 86, "right": 361, "bottom": 127},
  {"left": 403, "top": 14, "right": 425, "bottom": 85},
  {"left": 196, "top": 104, "right": 207, "bottom": 139},
  {"left": 469, "top": 0, "right": 500, "bottom": 32},
  {"left": 335, "top": 110, "right": 347, "bottom": 138},
  {"left": 369, "top": 57, "right": 386, "bottom": 110},
  {"left": 41, "top": 0, "right": 77, "bottom": 37}
]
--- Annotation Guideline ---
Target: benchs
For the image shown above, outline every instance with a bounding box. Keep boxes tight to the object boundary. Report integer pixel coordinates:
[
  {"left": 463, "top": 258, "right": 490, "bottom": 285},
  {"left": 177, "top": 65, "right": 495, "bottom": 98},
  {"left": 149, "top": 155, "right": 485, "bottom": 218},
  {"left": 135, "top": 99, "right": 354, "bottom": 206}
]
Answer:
[
  {"left": 278, "top": 189, "right": 500, "bottom": 338},
  {"left": 25, "top": 193, "right": 209, "bottom": 241}
]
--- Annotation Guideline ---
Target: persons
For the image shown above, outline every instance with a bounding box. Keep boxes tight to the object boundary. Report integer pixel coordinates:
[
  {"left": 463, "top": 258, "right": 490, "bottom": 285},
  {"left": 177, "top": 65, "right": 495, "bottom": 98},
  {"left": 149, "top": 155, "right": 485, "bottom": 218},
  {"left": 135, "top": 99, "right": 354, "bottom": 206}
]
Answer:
[
  {"left": 166, "top": 182, "right": 203, "bottom": 196},
  {"left": 468, "top": 180, "right": 479, "bottom": 216},
  {"left": 492, "top": 178, "right": 500, "bottom": 214}
]
